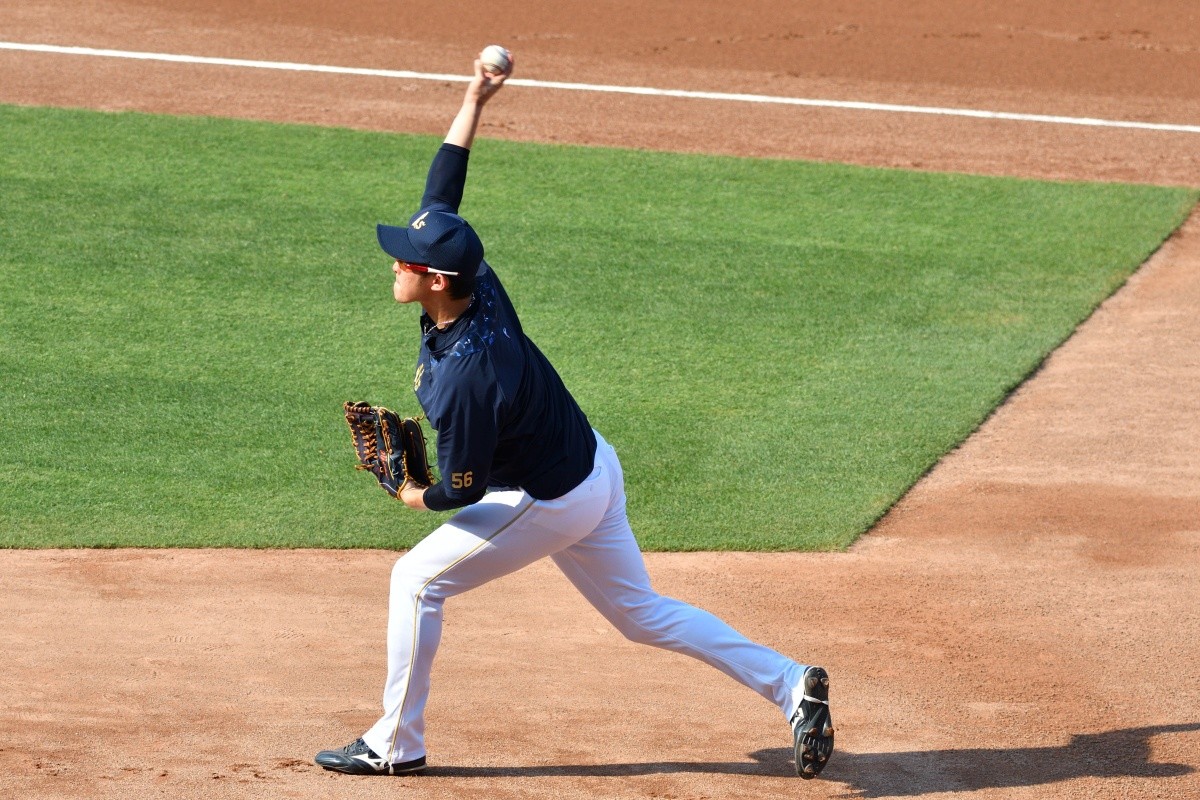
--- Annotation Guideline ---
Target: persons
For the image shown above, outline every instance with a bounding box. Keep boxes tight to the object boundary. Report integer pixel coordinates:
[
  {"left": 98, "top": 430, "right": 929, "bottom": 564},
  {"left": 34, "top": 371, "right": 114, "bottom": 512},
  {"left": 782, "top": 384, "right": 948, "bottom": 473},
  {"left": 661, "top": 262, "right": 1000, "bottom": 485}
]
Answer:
[{"left": 314, "top": 48, "right": 835, "bottom": 781}]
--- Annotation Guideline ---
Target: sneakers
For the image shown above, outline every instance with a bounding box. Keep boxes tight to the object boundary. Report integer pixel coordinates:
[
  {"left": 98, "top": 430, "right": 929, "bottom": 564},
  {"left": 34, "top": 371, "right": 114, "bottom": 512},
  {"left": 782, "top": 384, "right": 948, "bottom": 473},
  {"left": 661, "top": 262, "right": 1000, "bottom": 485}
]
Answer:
[
  {"left": 315, "top": 738, "right": 427, "bottom": 775},
  {"left": 792, "top": 666, "right": 835, "bottom": 779}
]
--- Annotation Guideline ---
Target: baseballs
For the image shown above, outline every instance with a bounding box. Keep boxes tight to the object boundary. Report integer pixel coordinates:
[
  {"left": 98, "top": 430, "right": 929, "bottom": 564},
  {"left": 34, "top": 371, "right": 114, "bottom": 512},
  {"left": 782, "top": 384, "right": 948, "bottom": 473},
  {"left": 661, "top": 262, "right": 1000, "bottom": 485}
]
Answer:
[{"left": 480, "top": 44, "right": 509, "bottom": 75}]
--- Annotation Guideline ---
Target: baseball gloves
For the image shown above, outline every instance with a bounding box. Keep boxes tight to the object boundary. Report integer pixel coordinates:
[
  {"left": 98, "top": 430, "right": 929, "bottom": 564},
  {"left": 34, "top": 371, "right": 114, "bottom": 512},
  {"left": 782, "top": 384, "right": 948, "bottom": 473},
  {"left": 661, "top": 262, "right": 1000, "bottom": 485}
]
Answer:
[{"left": 342, "top": 398, "right": 441, "bottom": 506}]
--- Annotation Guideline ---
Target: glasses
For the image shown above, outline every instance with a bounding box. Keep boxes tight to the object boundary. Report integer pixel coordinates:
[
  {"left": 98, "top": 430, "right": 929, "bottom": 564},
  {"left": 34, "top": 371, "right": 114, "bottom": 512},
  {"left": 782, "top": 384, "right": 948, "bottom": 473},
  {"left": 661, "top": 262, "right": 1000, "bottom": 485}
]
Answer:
[{"left": 396, "top": 257, "right": 460, "bottom": 277}]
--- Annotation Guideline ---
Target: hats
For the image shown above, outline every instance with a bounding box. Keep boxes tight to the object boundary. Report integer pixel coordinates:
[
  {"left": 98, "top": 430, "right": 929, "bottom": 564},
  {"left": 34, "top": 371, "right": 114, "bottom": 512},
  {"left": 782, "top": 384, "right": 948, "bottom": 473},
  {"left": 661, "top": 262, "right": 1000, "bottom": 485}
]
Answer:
[{"left": 376, "top": 211, "right": 484, "bottom": 277}]
